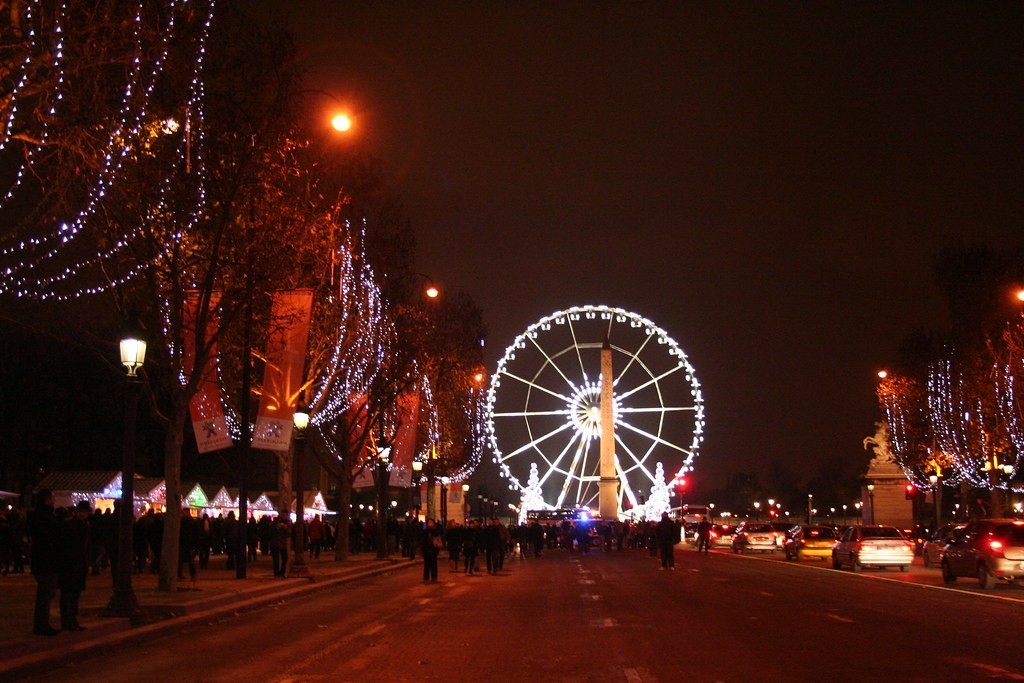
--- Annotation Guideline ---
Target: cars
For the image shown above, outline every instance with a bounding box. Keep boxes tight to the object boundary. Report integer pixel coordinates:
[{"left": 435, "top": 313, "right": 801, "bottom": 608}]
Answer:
[
  {"left": 940, "top": 517, "right": 1024, "bottom": 587},
  {"left": 696, "top": 525, "right": 733, "bottom": 546},
  {"left": 733, "top": 522, "right": 777, "bottom": 554},
  {"left": 781, "top": 525, "right": 837, "bottom": 561},
  {"left": 896, "top": 527, "right": 925, "bottom": 555},
  {"left": 769, "top": 521, "right": 798, "bottom": 548},
  {"left": 831, "top": 526, "right": 914, "bottom": 573},
  {"left": 922, "top": 523, "right": 973, "bottom": 567}
]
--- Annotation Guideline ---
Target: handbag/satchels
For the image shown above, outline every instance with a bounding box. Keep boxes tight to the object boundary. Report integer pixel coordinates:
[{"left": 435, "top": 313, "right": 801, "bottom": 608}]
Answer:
[{"left": 432, "top": 536, "right": 443, "bottom": 549}]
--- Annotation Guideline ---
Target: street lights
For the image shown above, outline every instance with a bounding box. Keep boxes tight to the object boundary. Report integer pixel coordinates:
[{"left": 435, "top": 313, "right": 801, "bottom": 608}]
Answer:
[
  {"left": 483, "top": 496, "right": 488, "bottom": 527},
  {"left": 867, "top": 484, "right": 875, "bottom": 526},
  {"left": 508, "top": 504, "right": 519, "bottom": 525},
  {"left": 831, "top": 507, "right": 836, "bottom": 525},
  {"left": 290, "top": 409, "right": 312, "bottom": 576},
  {"left": 494, "top": 500, "right": 499, "bottom": 519},
  {"left": 479, "top": 492, "right": 483, "bottom": 527},
  {"left": 462, "top": 482, "right": 470, "bottom": 527},
  {"left": 412, "top": 460, "right": 423, "bottom": 520},
  {"left": 842, "top": 504, "right": 847, "bottom": 525},
  {"left": 441, "top": 475, "right": 451, "bottom": 539},
  {"left": 855, "top": 503, "right": 860, "bottom": 524},
  {"left": 232, "top": 98, "right": 353, "bottom": 578},
  {"left": 109, "top": 334, "right": 149, "bottom": 614},
  {"left": 377, "top": 436, "right": 393, "bottom": 559},
  {"left": 754, "top": 501, "right": 761, "bottom": 522}
]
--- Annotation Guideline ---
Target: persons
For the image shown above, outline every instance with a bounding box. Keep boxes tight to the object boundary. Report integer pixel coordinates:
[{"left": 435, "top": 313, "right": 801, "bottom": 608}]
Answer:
[
  {"left": 696, "top": 517, "right": 712, "bottom": 554},
  {"left": 0, "top": 488, "right": 681, "bottom": 637}
]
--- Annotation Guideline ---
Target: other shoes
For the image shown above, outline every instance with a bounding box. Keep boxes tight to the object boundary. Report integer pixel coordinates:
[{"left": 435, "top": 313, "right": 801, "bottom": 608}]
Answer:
[
  {"left": 658, "top": 566, "right": 666, "bottom": 571},
  {"left": 33, "top": 628, "right": 57, "bottom": 635},
  {"left": 670, "top": 566, "right": 674, "bottom": 571},
  {"left": 63, "top": 623, "right": 87, "bottom": 630}
]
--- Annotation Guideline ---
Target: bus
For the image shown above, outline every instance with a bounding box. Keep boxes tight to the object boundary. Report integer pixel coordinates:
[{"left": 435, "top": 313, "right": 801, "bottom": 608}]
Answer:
[
  {"left": 667, "top": 504, "right": 710, "bottom": 524},
  {"left": 555, "top": 518, "right": 620, "bottom": 547},
  {"left": 526, "top": 508, "right": 593, "bottom": 526}
]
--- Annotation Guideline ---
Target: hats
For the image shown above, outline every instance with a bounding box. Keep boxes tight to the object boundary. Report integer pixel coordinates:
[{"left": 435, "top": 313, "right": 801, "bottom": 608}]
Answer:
[{"left": 75, "top": 500, "right": 92, "bottom": 513}]
